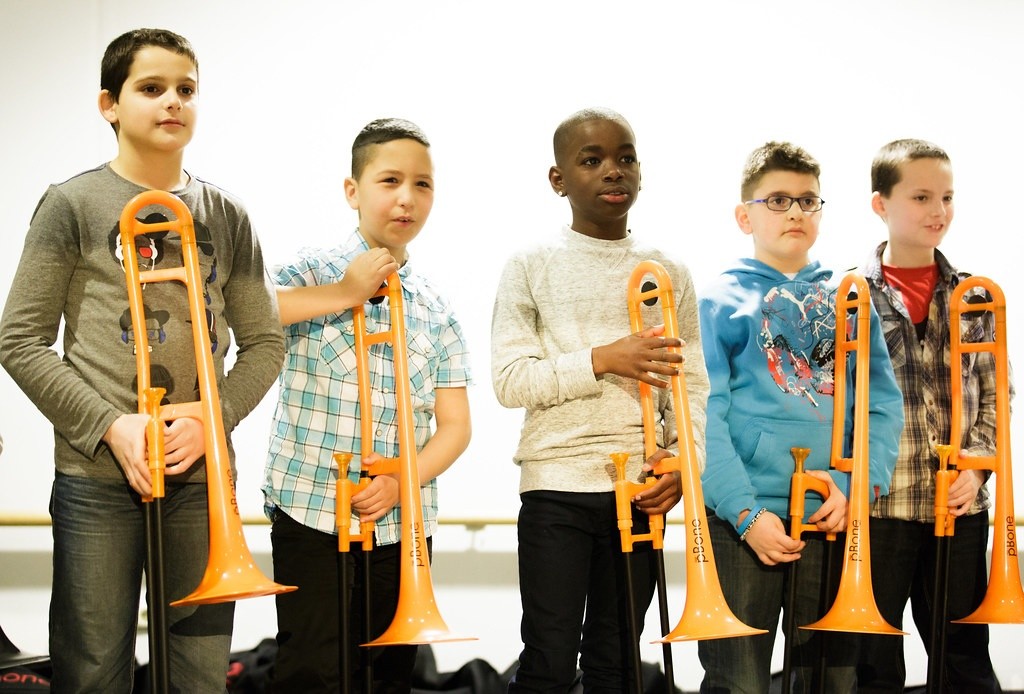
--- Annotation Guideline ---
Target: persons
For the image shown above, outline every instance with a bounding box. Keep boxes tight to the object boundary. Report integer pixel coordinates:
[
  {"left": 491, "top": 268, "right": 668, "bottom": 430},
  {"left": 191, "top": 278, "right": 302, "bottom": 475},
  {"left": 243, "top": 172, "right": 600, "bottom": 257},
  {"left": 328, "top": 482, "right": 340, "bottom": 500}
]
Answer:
[
  {"left": 490, "top": 107, "right": 710, "bottom": 694},
  {"left": 857, "top": 136, "right": 1015, "bottom": 694},
  {"left": 0, "top": 29, "right": 284, "bottom": 694},
  {"left": 263, "top": 117, "right": 470, "bottom": 693},
  {"left": 695, "top": 141, "right": 903, "bottom": 694}
]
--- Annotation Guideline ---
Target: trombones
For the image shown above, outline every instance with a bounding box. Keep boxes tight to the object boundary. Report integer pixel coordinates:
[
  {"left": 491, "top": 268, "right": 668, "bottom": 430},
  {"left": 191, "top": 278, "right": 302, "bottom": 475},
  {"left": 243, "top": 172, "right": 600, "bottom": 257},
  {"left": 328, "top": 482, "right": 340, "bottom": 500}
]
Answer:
[
  {"left": 788, "top": 275, "right": 903, "bottom": 693},
  {"left": 929, "top": 277, "right": 1024, "bottom": 693},
  {"left": 118, "top": 189, "right": 299, "bottom": 694},
  {"left": 333, "top": 268, "right": 482, "bottom": 693},
  {"left": 613, "top": 261, "right": 769, "bottom": 693}
]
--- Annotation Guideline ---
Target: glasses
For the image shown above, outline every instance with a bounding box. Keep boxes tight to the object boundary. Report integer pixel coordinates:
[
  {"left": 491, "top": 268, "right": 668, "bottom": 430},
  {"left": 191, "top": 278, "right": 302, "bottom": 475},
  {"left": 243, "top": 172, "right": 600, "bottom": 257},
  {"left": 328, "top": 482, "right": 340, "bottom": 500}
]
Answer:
[{"left": 745, "top": 195, "right": 825, "bottom": 213}]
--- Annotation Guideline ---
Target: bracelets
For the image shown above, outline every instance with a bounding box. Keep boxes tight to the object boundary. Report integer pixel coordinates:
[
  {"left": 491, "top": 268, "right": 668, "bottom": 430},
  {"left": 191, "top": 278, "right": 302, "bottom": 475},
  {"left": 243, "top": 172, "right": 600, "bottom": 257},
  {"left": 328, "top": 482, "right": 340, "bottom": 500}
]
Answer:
[{"left": 736, "top": 504, "right": 767, "bottom": 542}]
